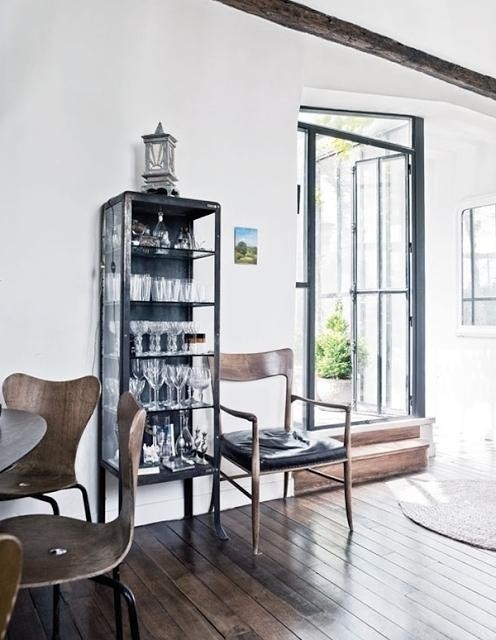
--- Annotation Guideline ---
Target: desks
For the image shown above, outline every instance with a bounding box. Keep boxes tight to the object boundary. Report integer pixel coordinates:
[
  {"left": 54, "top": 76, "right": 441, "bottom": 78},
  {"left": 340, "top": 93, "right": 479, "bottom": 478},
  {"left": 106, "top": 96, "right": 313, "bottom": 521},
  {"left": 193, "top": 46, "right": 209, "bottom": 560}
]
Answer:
[{"left": 0, "top": 409, "right": 47, "bottom": 473}]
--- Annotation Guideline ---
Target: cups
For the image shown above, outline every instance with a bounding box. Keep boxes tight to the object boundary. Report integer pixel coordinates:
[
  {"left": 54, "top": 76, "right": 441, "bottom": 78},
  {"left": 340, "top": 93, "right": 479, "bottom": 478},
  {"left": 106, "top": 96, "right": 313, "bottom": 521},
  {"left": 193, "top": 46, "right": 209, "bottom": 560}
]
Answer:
[{"left": 107, "top": 271, "right": 207, "bottom": 304}]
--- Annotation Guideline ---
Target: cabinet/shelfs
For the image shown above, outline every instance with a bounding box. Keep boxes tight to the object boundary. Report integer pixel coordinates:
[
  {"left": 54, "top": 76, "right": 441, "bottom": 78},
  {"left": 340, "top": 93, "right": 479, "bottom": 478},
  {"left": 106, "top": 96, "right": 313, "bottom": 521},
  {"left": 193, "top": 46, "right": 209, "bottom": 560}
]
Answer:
[{"left": 100, "top": 192, "right": 232, "bottom": 541}]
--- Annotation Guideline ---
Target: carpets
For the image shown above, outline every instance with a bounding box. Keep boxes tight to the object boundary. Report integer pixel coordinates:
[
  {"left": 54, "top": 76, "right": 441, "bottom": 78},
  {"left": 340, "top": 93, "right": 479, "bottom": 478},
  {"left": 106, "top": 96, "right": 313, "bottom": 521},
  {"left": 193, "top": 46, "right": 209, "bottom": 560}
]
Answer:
[{"left": 400, "top": 445, "right": 496, "bottom": 553}]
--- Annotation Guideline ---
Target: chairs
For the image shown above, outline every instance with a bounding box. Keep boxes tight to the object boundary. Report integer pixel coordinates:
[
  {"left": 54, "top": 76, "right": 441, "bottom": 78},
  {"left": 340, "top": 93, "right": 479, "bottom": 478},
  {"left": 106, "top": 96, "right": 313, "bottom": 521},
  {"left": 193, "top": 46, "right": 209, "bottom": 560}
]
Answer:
[
  {"left": 0, "top": 532, "right": 23, "bottom": 639},
  {"left": 2, "top": 372, "right": 101, "bottom": 526},
  {"left": 1, "top": 390, "right": 154, "bottom": 638},
  {"left": 207, "top": 346, "right": 355, "bottom": 557}
]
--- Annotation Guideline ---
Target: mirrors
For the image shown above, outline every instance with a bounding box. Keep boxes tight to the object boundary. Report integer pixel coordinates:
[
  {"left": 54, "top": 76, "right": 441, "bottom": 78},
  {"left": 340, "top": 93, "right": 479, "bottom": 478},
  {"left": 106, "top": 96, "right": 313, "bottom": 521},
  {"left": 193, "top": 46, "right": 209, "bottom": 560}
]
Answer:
[{"left": 455, "top": 192, "right": 496, "bottom": 337}]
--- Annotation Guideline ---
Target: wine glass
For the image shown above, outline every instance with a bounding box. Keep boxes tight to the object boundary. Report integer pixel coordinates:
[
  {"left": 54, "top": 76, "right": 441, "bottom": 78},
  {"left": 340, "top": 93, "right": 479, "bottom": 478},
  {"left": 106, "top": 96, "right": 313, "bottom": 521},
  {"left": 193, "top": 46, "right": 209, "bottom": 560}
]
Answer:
[
  {"left": 109, "top": 319, "right": 197, "bottom": 357},
  {"left": 103, "top": 359, "right": 212, "bottom": 411}
]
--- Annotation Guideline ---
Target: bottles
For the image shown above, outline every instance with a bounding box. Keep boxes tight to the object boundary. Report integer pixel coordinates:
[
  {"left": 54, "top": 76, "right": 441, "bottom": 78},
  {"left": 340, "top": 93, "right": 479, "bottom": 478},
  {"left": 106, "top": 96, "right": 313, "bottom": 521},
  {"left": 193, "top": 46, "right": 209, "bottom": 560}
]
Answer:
[
  {"left": 153, "top": 208, "right": 171, "bottom": 247},
  {"left": 176, "top": 226, "right": 198, "bottom": 250},
  {"left": 150, "top": 417, "right": 195, "bottom": 464}
]
